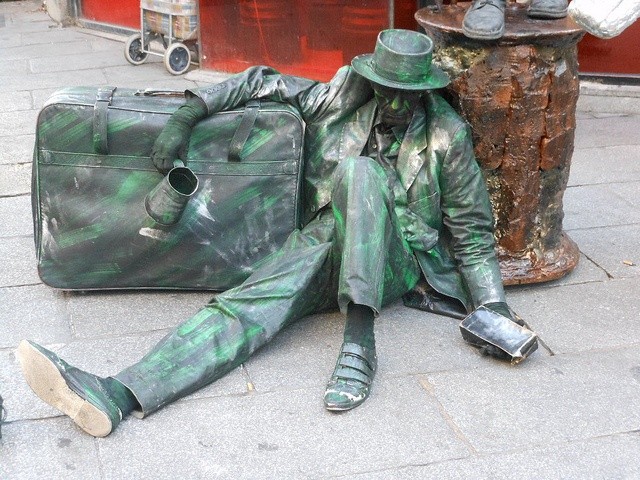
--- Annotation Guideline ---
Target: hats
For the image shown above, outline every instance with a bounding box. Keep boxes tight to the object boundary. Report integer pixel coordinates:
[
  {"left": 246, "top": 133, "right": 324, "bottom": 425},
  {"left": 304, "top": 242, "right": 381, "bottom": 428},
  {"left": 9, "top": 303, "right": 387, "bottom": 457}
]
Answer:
[{"left": 351, "top": 28, "right": 450, "bottom": 90}]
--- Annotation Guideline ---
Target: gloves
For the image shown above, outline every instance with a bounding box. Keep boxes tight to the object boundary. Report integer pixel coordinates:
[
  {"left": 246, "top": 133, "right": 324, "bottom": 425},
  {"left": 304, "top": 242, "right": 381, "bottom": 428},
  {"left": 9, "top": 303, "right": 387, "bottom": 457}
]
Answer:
[
  {"left": 479, "top": 304, "right": 525, "bottom": 357},
  {"left": 151, "top": 101, "right": 201, "bottom": 174}
]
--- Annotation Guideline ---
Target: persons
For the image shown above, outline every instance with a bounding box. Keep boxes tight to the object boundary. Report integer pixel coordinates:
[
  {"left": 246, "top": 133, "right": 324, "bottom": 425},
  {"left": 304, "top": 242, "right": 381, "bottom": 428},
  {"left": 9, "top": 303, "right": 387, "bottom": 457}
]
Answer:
[
  {"left": 19, "top": 29, "right": 538, "bottom": 437},
  {"left": 461, "top": 0, "right": 570, "bottom": 42}
]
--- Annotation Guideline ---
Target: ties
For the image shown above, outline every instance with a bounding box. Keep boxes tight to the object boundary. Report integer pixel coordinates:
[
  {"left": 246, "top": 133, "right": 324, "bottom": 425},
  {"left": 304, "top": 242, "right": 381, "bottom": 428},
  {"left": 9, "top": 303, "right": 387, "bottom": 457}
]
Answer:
[{"left": 374, "top": 128, "right": 439, "bottom": 250}]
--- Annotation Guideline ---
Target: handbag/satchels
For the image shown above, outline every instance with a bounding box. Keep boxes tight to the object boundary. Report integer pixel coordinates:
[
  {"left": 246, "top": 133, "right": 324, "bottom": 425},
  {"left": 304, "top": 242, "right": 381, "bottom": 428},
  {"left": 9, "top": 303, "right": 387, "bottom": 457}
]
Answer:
[{"left": 31, "top": 85, "right": 306, "bottom": 290}]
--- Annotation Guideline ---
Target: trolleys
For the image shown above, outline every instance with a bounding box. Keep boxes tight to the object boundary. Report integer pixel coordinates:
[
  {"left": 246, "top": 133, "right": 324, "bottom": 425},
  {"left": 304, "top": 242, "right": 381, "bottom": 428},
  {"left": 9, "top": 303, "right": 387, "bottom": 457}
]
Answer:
[{"left": 124, "top": 1, "right": 199, "bottom": 75}]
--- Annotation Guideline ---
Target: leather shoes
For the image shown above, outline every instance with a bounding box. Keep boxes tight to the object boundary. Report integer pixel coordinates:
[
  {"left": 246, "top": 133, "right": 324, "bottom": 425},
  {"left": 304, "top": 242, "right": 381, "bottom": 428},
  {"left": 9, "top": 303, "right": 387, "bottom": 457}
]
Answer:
[
  {"left": 18, "top": 340, "right": 122, "bottom": 437},
  {"left": 324, "top": 342, "right": 377, "bottom": 411},
  {"left": 462, "top": 0, "right": 504, "bottom": 39},
  {"left": 526, "top": 1, "right": 569, "bottom": 17}
]
